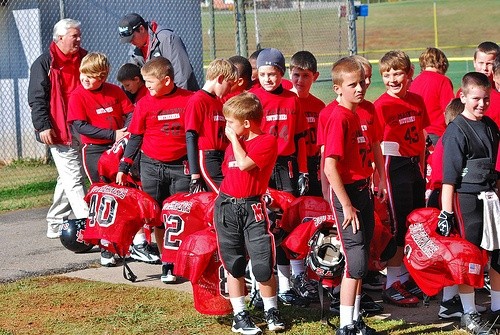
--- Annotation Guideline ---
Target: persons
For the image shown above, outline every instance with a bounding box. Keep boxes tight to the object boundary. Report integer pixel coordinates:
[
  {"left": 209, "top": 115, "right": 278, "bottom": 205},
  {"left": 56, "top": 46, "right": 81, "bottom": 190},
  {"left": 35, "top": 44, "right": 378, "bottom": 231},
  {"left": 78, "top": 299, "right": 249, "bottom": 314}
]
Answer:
[
  {"left": 72, "top": 14, "right": 500, "bottom": 335},
  {"left": 28, "top": 18, "right": 87, "bottom": 239}
]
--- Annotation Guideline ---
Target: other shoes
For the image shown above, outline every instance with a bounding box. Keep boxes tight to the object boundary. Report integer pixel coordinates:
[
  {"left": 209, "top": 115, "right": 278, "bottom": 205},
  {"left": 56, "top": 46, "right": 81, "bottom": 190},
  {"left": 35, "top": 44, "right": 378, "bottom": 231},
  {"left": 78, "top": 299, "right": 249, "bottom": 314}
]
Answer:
[{"left": 245, "top": 270, "right": 254, "bottom": 283}]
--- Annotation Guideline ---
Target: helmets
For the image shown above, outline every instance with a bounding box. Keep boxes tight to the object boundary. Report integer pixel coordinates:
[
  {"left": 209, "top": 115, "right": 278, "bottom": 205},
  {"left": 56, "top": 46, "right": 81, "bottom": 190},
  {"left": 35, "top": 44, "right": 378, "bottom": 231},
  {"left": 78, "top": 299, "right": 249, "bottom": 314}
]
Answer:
[
  {"left": 61, "top": 218, "right": 96, "bottom": 252},
  {"left": 305, "top": 229, "right": 344, "bottom": 280}
]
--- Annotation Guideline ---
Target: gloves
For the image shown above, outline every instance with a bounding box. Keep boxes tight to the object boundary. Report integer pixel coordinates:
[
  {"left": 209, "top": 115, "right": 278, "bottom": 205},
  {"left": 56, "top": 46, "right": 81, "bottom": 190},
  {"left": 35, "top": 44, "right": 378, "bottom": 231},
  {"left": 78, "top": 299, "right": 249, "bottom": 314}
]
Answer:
[
  {"left": 296, "top": 173, "right": 310, "bottom": 197},
  {"left": 435, "top": 210, "right": 454, "bottom": 238},
  {"left": 187, "top": 178, "right": 205, "bottom": 193}
]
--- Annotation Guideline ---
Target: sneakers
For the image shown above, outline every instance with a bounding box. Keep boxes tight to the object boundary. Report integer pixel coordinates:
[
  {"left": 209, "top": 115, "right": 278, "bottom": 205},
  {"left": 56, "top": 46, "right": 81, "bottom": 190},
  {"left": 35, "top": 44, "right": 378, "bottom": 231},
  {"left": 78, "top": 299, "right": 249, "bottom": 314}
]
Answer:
[
  {"left": 461, "top": 311, "right": 497, "bottom": 335},
  {"left": 487, "top": 308, "right": 500, "bottom": 328},
  {"left": 249, "top": 290, "right": 265, "bottom": 309},
  {"left": 231, "top": 310, "right": 263, "bottom": 335},
  {"left": 100, "top": 248, "right": 117, "bottom": 266},
  {"left": 329, "top": 289, "right": 383, "bottom": 316},
  {"left": 363, "top": 270, "right": 387, "bottom": 291},
  {"left": 47, "top": 221, "right": 64, "bottom": 239},
  {"left": 402, "top": 280, "right": 438, "bottom": 300},
  {"left": 161, "top": 263, "right": 177, "bottom": 283},
  {"left": 382, "top": 280, "right": 418, "bottom": 307},
  {"left": 264, "top": 308, "right": 286, "bottom": 331},
  {"left": 279, "top": 291, "right": 310, "bottom": 308},
  {"left": 438, "top": 295, "right": 486, "bottom": 322},
  {"left": 291, "top": 272, "right": 329, "bottom": 300},
  {"left": 130, "top": 242, "right": 161, "bottom": 264},
  {"left": 335, "top": 316, "right": 376, "bottom": 335}
]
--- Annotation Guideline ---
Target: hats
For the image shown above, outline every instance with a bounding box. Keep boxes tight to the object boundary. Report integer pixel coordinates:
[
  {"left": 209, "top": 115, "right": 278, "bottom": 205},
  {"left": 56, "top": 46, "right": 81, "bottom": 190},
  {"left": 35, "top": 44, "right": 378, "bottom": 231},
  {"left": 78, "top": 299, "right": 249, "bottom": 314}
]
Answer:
[
  {"left": 257, "top": 48, "right": 285, "bottom": 77},
  {"left": 118, "top": 13, "right": 145, "bottom": 44}
]
